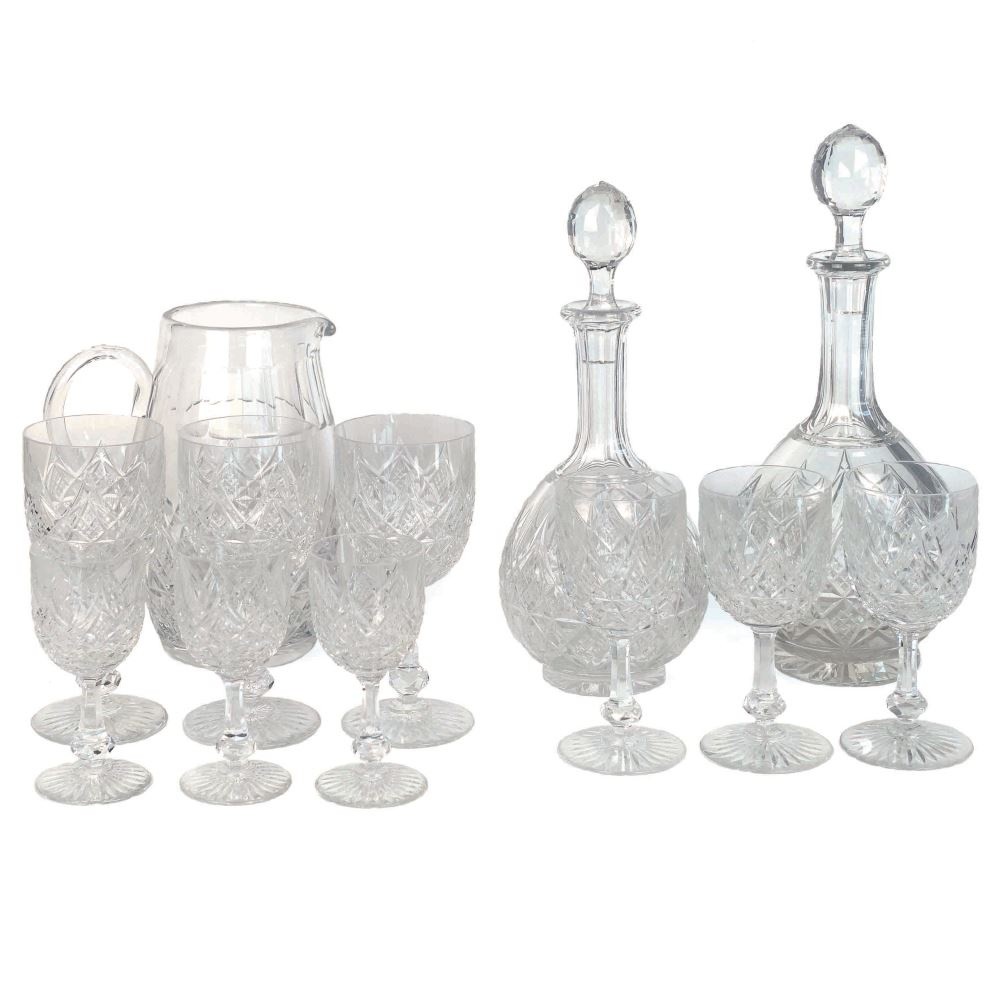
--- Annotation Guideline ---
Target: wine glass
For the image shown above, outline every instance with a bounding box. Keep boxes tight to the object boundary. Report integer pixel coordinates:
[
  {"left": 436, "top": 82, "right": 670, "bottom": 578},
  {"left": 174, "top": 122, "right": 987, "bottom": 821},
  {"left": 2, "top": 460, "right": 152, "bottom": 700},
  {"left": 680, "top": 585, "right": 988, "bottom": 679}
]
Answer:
[
  {"left": 553, "top": 471, "right": 687, "bottom": 776},
  {"left": 21, "top": 414, "right": 169, "bottom": 804},
  {"left": 147, "top": 415, "right": 320, "bottom": 806},
  {"left": 333, "top": 413, "right": 476, "bottom": 749},
  {"left": 698, "top": 465, "right": 832, "bottom": 775},
  {"left": 840, "top": 461, "right": 979, "bottom": 771},
  {"left": 308, "top": 534, "right": 429, "bottom": 809}
]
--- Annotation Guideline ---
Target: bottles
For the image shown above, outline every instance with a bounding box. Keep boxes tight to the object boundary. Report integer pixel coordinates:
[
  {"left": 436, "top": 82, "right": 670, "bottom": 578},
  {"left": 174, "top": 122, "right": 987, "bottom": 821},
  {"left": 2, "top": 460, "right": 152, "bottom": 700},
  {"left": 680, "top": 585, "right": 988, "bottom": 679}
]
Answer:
[
  {"left": 738, "top": 124, "right": 950, "bottom": 687},
  {"left": 498, "top": 180, "right": 709, "bottom": 698}
]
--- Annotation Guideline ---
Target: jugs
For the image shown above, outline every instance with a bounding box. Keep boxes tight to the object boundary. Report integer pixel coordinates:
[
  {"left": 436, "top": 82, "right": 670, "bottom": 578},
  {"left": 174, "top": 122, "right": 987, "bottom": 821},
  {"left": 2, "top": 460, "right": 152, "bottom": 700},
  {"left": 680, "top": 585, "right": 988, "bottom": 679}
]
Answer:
[{"left": 42, "top": 300, "right": 337, "bottom": 668}]
